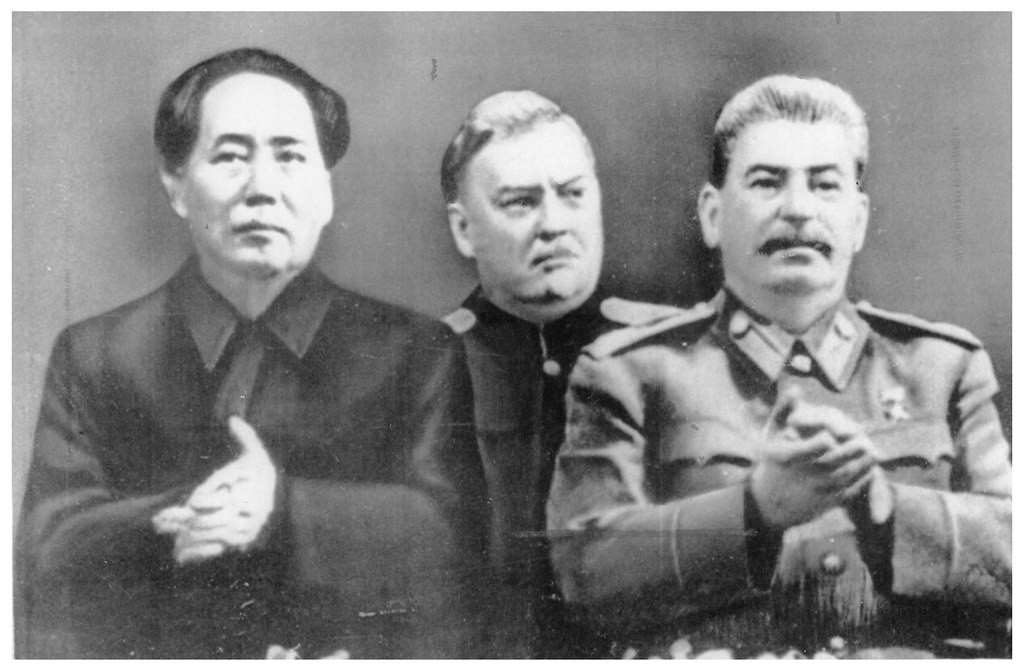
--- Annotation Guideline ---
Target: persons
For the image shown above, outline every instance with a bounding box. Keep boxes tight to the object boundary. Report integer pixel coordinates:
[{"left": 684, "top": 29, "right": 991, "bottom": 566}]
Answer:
[
  {"left": 12, "top": 48, "right": 493, "bottom": 660},
  {"left": 435, "top": 89, "right": 667, "bottom": 660},
  {"left": 544, "top": 75, "right": 1012, "bottom": 660}
]
